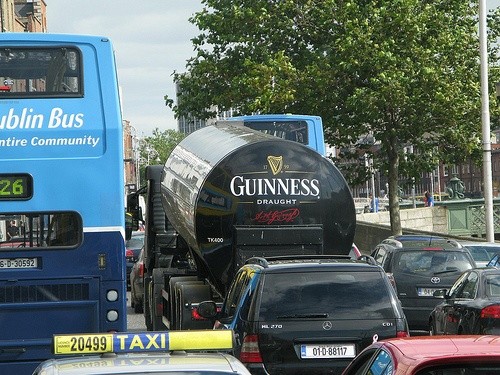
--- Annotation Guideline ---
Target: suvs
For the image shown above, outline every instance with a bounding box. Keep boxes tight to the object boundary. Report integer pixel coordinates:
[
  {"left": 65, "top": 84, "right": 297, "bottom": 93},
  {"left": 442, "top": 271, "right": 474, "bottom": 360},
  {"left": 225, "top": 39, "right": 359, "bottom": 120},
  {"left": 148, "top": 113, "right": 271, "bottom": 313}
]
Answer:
[
  {"left": 197, "top": 253, "right": 410, "bottom": 375},
  {"left": 368, "top": 232, "right": 477, "bottom": 336}
]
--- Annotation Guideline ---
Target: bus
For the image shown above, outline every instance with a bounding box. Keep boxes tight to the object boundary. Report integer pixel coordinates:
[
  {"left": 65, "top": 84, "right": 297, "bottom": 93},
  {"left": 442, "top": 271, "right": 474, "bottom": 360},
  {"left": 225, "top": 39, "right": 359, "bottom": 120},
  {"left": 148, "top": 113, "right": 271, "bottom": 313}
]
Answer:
[
  {"left": 220, "top": 114, "right": 324, "bottom": 158},
  {"left": 0, "top": 30, "right": 128, "bottom": 375}
]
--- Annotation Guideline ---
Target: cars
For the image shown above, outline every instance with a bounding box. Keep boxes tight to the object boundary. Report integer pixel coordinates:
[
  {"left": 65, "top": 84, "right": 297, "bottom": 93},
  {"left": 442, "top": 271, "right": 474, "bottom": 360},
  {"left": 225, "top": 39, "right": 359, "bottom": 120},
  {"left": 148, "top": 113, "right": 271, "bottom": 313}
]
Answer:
[
  {"left": 31, "top": 331, "right": 250, "bottom": 375},
  {"left": 125, "top": 194, "right": 146, "bottom": 314},
  {"left": 342, "top": 336, "right": 500, "bottom": 375},
  {"left": 451, "top": 239, "right": 500, "bottom": 267},
  {"left": 428, "top": 268, "right": 500, "bottom": 337}
]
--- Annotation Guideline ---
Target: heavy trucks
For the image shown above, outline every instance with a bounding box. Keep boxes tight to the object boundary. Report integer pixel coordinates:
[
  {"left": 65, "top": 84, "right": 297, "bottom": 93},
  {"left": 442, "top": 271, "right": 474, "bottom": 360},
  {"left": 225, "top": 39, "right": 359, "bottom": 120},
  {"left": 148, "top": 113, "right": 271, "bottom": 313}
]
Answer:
[{"left": 130, "top": 122, "right": 356, "bottom": 332}]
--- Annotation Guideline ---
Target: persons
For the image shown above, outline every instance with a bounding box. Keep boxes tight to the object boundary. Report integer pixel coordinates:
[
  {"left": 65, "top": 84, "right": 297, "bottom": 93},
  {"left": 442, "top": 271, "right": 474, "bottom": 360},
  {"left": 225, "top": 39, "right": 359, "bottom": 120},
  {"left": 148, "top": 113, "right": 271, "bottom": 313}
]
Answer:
[
  {"left": 424, "top": 191, "right": 434, "bottom": 207},
  {"left": 369, "top": 192, "right": 379, "bottom": 213}
]
